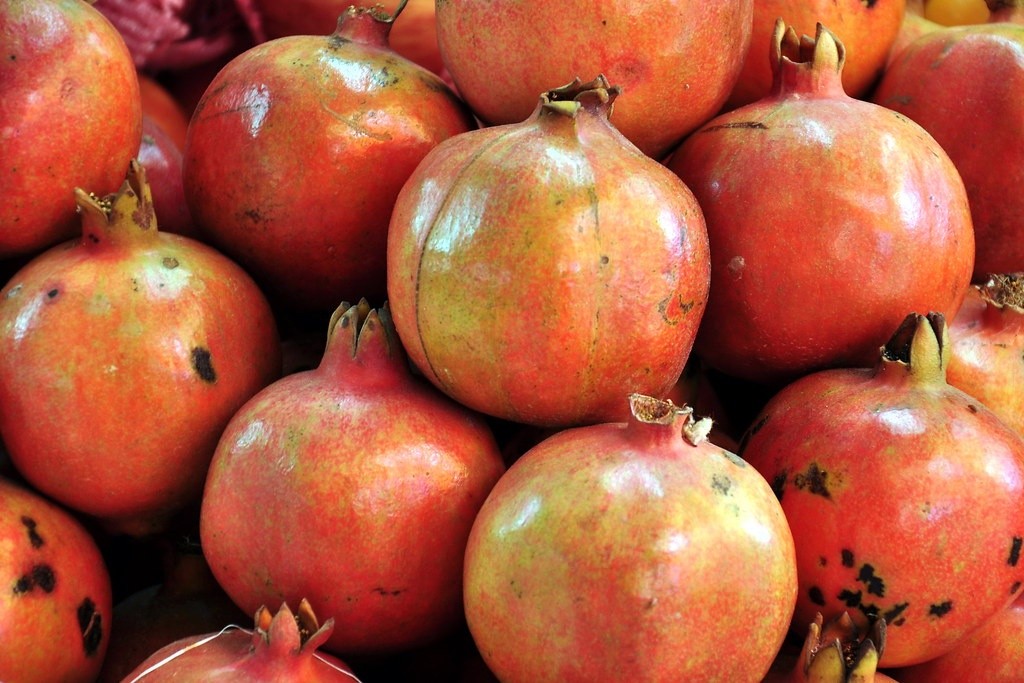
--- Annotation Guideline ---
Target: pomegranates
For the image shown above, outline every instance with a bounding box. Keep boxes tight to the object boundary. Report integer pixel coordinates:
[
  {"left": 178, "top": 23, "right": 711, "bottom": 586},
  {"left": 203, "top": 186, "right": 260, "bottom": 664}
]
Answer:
[{"left": 0, "top": 0, "right": 1024, "bottom": 683}]
